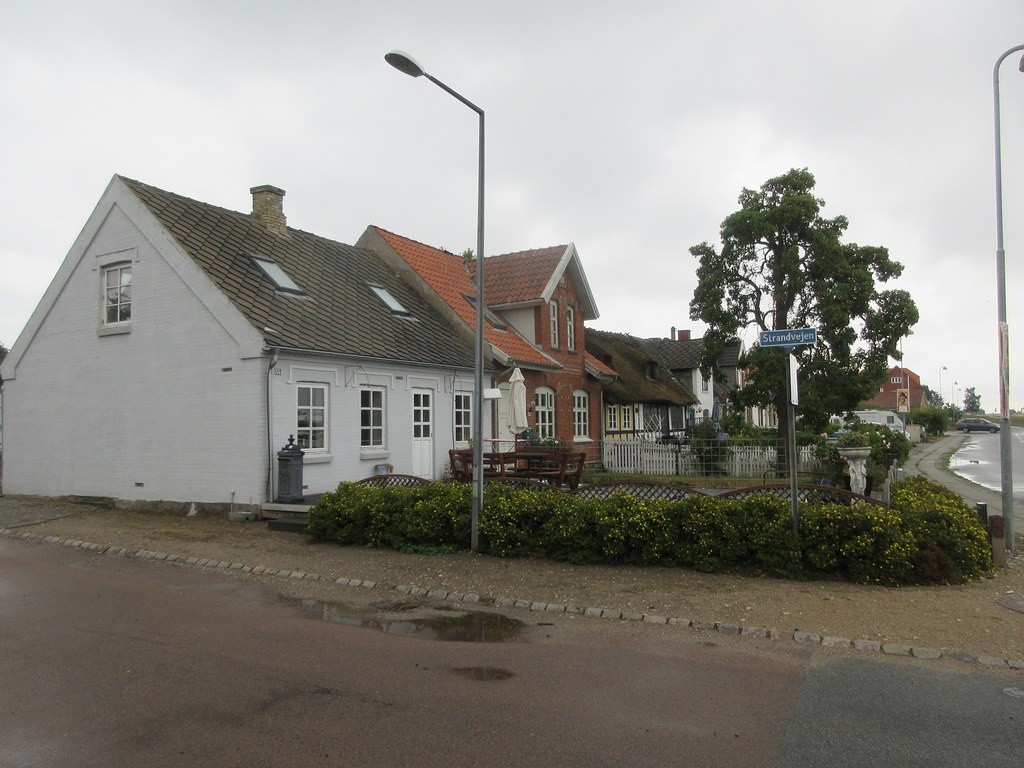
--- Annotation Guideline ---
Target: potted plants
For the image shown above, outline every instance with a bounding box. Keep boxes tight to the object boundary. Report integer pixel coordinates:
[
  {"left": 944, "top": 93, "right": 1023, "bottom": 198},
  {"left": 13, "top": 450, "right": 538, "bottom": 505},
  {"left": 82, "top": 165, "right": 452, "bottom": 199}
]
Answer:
[{"left": 836, "top": 431, "right": 873, "bottom": 459}]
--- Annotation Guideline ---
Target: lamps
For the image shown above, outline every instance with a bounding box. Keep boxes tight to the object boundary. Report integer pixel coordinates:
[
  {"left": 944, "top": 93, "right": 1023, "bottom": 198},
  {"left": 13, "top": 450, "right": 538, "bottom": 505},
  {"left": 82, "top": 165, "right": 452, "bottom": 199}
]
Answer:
[{"left": 526, "top": 401, "right": 535, "bottom": 412}]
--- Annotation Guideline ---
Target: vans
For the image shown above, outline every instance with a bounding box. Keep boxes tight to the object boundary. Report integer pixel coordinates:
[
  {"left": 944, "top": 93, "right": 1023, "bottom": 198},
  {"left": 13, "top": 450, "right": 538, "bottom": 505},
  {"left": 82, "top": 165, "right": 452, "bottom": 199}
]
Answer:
[{"left": 827, "top": 409, "right": 911, "bottom": 449}]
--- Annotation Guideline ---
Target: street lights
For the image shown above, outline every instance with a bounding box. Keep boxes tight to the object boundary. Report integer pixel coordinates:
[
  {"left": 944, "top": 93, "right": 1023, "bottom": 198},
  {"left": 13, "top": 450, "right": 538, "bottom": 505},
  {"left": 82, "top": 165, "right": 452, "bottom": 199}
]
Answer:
[
  {"left": 938, "top": 365, "right": 947, "bottom": 399},
  {"left": 383, "top": 48, "right": 486, "bottom": 553},
  {"left": 991, "top": 43, "right": 1024, "bottom": 557},
  {"left": 951, "top": 380, "right": 962, "bottom": 409}
]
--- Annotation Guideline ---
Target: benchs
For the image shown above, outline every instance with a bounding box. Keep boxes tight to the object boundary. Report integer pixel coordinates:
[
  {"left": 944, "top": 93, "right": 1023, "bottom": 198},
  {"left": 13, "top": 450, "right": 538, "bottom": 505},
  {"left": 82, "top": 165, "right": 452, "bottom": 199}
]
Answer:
[{"left": 447, "top": 449, "right": 586, "bottom": 491}]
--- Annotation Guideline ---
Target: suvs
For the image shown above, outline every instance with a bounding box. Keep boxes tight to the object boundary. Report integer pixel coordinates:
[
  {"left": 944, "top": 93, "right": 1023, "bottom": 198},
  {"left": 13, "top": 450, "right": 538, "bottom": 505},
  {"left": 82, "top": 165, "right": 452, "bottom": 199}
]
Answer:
[{"left": 956, "top": 418, "right": 1001, "bottom": 434}]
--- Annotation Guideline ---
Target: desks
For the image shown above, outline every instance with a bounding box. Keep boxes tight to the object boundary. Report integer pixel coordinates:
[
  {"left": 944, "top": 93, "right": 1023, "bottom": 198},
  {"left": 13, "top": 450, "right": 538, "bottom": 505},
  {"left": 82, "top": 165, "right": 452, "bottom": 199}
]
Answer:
[{"left": 507, "top": 452, "right": 556, "bottom": 490}]
